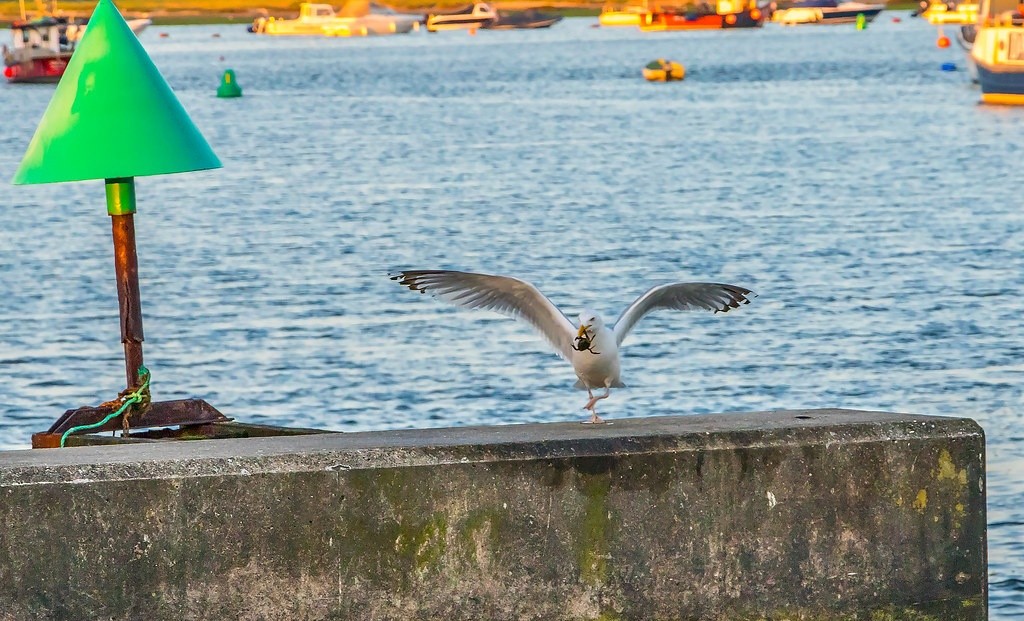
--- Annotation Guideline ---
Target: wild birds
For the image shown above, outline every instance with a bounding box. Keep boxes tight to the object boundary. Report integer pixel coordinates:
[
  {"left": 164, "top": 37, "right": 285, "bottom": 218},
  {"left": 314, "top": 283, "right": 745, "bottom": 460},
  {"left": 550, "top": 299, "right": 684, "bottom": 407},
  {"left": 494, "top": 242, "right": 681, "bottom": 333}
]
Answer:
[{"left": 388, "top": 270, "right": 758, "bottom": 425}]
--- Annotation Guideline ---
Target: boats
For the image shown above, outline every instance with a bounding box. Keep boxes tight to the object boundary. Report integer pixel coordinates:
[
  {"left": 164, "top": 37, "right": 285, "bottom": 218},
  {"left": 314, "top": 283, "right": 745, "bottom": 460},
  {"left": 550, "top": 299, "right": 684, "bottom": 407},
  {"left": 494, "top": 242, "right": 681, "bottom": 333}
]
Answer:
[
  {"left": 5, "top": 11, "right": 153, "bottom": 85},
  {"left": 595, "top": 0, "right": 1024, "bottom": 119},
  {"left": 249, "top": 0, "right": 562, "bottom": 38}
]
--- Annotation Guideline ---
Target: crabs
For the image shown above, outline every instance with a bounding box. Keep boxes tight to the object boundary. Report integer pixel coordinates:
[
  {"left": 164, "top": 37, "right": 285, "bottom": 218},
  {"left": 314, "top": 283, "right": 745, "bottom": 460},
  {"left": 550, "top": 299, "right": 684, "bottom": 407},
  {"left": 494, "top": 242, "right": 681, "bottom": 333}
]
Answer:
[{"left": 571, "top": 329, "right": 602, "bottom": 356}]
